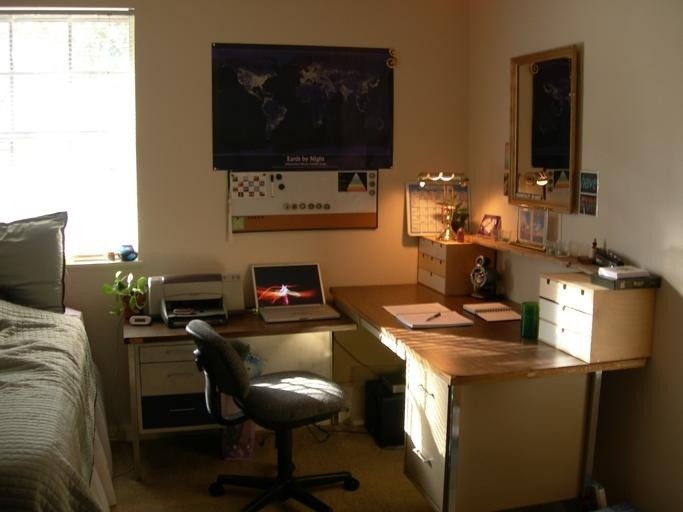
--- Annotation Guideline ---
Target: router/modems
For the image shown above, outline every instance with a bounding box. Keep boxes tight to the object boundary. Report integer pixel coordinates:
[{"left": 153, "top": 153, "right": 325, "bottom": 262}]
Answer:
[{"left": 381, "top": 372, "right": 405, "bottom": 394}]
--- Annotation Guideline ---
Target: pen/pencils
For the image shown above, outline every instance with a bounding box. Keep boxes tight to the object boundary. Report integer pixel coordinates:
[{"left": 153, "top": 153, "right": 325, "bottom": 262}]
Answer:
[{"left": 426, "top": 312, "right": 441, "bottom": 321}]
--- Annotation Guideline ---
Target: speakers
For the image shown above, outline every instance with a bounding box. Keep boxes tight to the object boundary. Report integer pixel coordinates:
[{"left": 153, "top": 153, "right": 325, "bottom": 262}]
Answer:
[{"left": 364, "top": 379, "right": 404, "bottom": 448}]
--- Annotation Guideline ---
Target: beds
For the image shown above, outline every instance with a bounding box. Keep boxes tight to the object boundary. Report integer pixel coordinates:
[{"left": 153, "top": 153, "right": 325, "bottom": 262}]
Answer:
[{"left": 0, "top": 297, "right": 118, "bottom": 512}]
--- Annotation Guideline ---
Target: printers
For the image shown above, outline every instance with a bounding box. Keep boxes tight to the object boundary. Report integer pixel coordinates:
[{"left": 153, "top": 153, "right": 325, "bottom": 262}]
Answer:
[{"left": 148, "top": 272, "right": 229, "bottom": 329}]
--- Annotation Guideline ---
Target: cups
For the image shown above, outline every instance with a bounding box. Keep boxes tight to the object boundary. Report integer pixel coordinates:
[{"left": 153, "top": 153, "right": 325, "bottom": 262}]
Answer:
[
  {"left": 544, "top": 238, "right": 580, "bottom": 258},
  {"left": 519, "top": 300, "right": 538, "bottom": 339}
]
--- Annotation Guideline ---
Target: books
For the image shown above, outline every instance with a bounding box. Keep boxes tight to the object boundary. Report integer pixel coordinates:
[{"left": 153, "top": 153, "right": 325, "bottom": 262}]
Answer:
[
  {"left": 382, "top": 301, "right": 474, "bottom": 329},
  {"left": 463, "top": 302, "right": 520, "bottom": 323},
  {"left": 591, "top": 264, "right": 662, "bottom": 291}
]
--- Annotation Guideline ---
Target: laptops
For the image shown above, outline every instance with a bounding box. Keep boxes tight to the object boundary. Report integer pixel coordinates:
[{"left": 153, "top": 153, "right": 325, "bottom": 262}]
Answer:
[{"left": 251, "top": 263, "right": 341, "bottom": 322}]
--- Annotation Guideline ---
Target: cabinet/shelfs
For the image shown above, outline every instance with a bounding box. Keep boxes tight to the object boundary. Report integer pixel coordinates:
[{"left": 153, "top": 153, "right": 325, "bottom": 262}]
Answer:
[
  {"left": 417, "top": 236, "right": 496, "bottom": 296},
  {"left": 538, "top": 273, "right": 658, "bottom": 365}
]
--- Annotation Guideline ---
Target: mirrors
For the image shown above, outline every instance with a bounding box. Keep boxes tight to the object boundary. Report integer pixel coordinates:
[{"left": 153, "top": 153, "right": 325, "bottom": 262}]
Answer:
[{"left": 509, "top": 42, "right": 580, "bottom": 214}]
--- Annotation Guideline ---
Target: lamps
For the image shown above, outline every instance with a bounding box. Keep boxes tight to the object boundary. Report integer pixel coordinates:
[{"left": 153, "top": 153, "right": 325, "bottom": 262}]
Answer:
[{"left": 419, "top": 172, "right": 468, "bottom": 241}]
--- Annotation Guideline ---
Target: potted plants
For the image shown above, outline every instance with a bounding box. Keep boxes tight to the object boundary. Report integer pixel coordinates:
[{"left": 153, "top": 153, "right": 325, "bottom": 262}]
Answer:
[{"left": 103, "top": 268, "right": 148, "bottom": 320}]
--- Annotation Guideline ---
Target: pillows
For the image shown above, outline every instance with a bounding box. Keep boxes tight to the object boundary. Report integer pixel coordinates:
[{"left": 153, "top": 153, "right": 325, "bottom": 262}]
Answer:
[{"left": 0, "top": 211, "right": 68, "bottom": 314}]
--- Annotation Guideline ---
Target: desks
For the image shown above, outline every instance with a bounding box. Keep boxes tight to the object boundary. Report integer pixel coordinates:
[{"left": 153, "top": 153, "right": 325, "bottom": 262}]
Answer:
[
  {"left": 123, "top": 303, "right": 358, "bottom": 481},
  {"left": 329, "top": 284, "right": 647, "bottom": 511}
]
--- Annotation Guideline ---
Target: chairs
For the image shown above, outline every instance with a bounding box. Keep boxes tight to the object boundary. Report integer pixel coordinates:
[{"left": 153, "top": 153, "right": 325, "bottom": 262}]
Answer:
[{"left": 184, "top": 319, "right": 360, "bottom": 512}]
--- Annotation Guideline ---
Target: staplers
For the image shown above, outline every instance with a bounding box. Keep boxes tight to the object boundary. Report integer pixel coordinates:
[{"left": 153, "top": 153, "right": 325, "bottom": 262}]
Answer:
[{"left": 592, "top": 248, "right": 624, "bottom": 267}]
